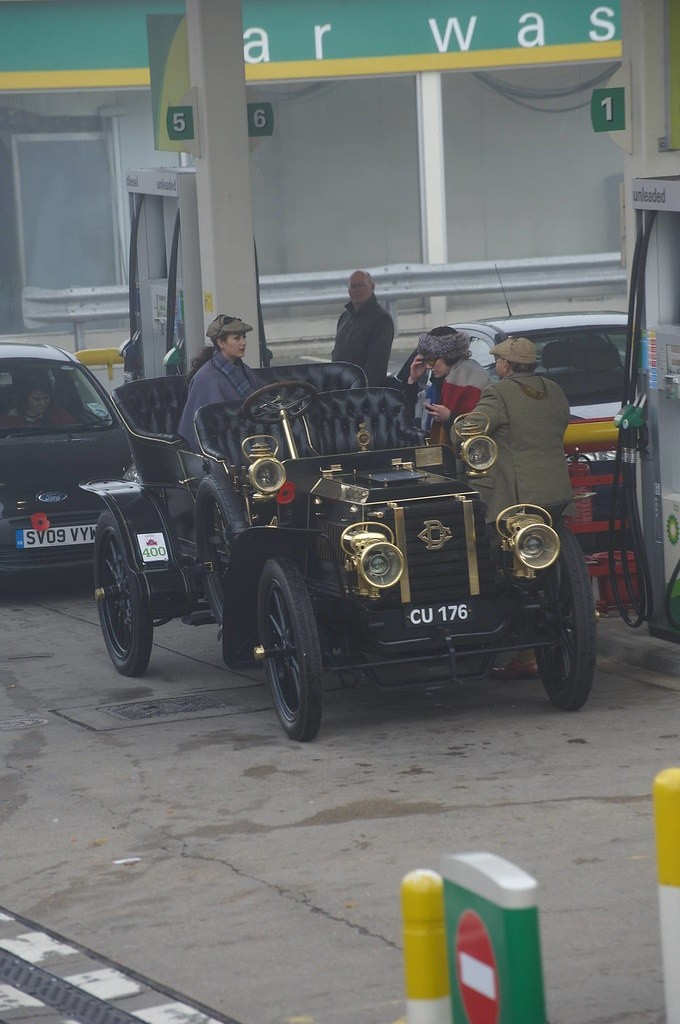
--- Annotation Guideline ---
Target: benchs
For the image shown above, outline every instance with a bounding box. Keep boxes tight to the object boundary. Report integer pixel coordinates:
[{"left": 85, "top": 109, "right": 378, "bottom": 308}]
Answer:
[{"left": 112, "top": 360, "right": 430, "bottom": 501}]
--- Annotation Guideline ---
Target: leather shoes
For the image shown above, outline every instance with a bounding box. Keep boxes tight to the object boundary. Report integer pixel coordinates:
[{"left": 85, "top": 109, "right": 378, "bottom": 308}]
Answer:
[{"left": 491, "top": 656, "right": 539, "bottom": 680}]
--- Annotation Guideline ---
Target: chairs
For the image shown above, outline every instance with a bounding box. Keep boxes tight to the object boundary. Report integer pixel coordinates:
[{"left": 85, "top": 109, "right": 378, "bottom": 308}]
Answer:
[
  {"left": 585, "top": 341, "right": 628, "bottom": 402},
  {"left": 542, "top": 341, "right": 595, "bottom": 406}
]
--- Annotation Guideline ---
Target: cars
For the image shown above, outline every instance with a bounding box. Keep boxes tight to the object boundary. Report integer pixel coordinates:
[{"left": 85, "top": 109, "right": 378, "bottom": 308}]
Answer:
[
  {"left": 397, "top": 310, "right": 635, "bottom": 462},
  {"left": 0, "top": 341, "right": 141, "bottom": 573},
  {"left": 77, "top": 360, "right": 598, "bottom": 743}
]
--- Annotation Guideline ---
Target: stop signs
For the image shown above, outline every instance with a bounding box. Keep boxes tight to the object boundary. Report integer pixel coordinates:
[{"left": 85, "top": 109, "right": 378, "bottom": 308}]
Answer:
[{"left": 455, "top": 910, "right": 501, "bottom": 1024}]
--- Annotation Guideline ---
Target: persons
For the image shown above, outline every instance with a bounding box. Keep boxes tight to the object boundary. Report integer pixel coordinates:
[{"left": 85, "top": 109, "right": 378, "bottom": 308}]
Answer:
[
  {"left": 406, "top": 326, "right": 490, "bottom": 456},
  {"left": 173, "top": 315, "right": 265, "bottom": 458},
  {"left": 0, "top": 374, "right": 76, "bottom": 427},
  {"left": 332, "top": 271, "right": 394, "bottom": 387},
  {"left": 450, "top": 338, "right": 572, "bottom": 682}
]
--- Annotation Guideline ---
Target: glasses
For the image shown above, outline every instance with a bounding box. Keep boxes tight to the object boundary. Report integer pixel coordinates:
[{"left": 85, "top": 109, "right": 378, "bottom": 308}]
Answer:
[
  {"left": 216, "top": 316, "right": 242, "bottom": 338},
  {"left": 422, "top": 356, "right": 441, "bottom": 365}
]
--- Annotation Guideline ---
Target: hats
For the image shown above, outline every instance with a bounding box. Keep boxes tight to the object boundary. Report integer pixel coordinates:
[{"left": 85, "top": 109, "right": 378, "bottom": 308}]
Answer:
[
  {"left": 418, "top": 326, "right": 471, "bottom": 359},
  {"left": 206, "top": 315, "right": 253, "bottom": 336},
  {"left": 489, "top": 336, "right": 536, "bottom": 366}
]
